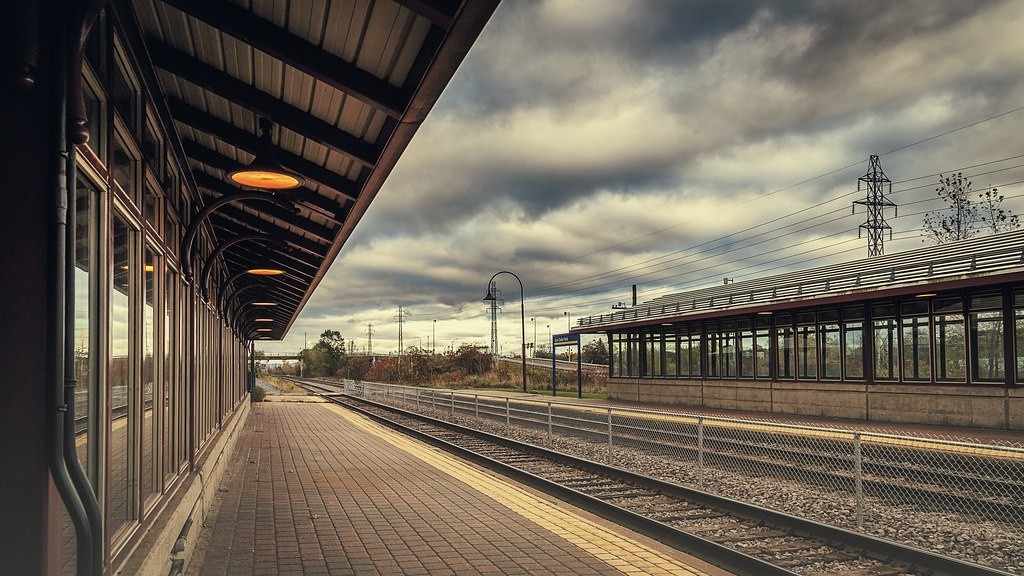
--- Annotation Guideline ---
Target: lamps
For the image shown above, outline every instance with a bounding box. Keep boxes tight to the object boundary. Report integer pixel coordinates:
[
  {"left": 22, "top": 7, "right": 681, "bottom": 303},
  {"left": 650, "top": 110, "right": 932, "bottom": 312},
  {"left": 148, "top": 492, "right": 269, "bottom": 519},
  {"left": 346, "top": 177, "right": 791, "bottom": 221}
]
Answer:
[
  {"left": 241, "top": 261, "right": 286, "bottom": 276},
  {"left": 250, "top": 295, "right": 279, "bottom": 338},
  {"left": 225, "top": 120, "right": 305, "bottom": 190}
]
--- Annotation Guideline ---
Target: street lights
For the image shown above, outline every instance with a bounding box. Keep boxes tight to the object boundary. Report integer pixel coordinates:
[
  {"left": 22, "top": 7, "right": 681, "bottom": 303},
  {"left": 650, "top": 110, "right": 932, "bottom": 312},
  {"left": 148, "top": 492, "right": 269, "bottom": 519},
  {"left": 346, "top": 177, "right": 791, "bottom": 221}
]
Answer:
[
  {"left": 417, "top": 336, "right": 422, "bottom": 352},
  {"left": 304, "top": 331, "right": 307, "bottom": 349},
  {"left": 530, "top": 317, "right": 537, "bottom": 358},
  {"left": 546, "top": 325, "right": 552, "bottom": 358},
  {"left": 451, "top": 338, "right": 458, "bottom": 355},
  {"left": 439, "top": 345, "right": 447, "bottom": 356},
  {"left": 564, "top": 311, "right": 571, "bottom": 361},
  {"left": 482, "top": 270, "right": 527, "bottom": 392},
  {"left": 499, "top": 342, "right": 507, "bottom": 356},
  {"left": 426, "top": 335, "right": 429, "bottom": 350},
  {"left": 432, "top": 319, "right": 436, "bottom": 354}
]
